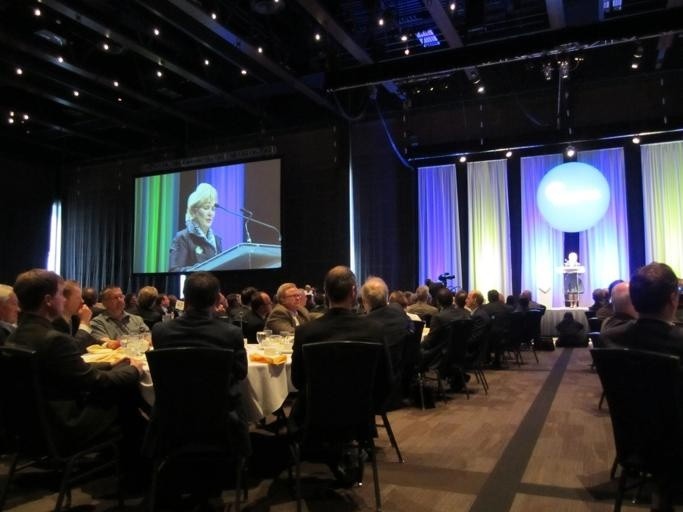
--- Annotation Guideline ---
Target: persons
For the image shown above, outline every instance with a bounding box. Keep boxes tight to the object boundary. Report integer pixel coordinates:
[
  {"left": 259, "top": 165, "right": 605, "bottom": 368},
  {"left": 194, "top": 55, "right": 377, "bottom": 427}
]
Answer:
[
  {"left": 165, "top": 183, "right": 225, "bottom": 273},
  {"left": 1, "top": 265, "right": 547, "bottom": 512},
  {"left": 588, "top": 262, "right": 683, "bottom": 512},
  {"left": 557, "top": 313, "right": 586, "bottom": 348},
  {"left": 561, "top": 251, "right": 585, "bottom": 308}
]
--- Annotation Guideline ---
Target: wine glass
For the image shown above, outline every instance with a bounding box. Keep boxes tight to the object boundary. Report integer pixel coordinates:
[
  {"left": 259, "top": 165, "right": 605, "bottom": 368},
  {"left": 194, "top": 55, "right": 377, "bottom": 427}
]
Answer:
[{"left": 116, "top": 323, "right": 297, "bottom": 366}]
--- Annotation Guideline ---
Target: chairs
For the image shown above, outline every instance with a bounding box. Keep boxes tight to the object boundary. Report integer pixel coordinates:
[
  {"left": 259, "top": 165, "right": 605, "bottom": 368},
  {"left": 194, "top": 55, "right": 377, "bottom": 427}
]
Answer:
[
  {"left": 140, "top": 345, "right": 252, "bottom": 510},
  {"left": 1, "top": 296, "right": 547, "bottom": 418},
  {"left": 584, "top": 309, "right": 681, "bottom": 511},
  {"left": 267, "top": 338, "right": 378, "bottom": 511},
  {"left": 2, "top": 347, "right": 127, "bottom": 511},
  {"left": 359, "top": 333, "right": 400, "bottom": 467}
]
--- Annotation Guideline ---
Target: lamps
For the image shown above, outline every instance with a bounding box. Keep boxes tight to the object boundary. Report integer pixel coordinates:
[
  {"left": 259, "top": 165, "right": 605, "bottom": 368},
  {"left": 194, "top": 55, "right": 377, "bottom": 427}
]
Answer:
[{"left": 533, "top": 56, "right": 610, "bottom": 232}]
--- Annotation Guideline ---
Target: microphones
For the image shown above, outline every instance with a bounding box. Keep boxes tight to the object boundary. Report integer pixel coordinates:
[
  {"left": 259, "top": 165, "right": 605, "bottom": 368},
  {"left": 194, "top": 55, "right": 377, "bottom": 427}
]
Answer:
[
  {"left": 240, "top": 208, "right": 253, "bottom": 241},
  {"left": 215, "top": 204, "right": 281, "bottom": 242}
]
